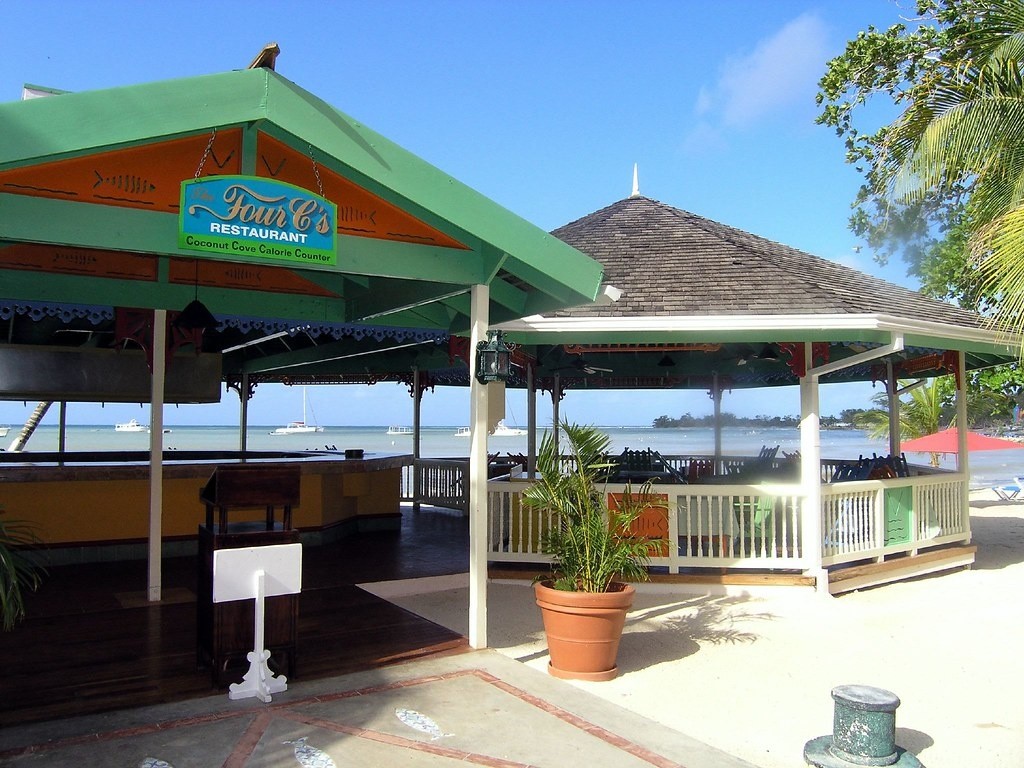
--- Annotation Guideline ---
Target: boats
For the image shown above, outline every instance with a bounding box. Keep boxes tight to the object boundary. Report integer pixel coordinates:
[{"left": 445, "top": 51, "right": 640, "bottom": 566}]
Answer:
[
  {"left": 386, "top": 426, "right": 414, "bottom": 435},
  {"left": 0, "top": 428, "right": 11, "bottom": 437},
  {"left": 115, "top": 419, "right": 149, "bottom": 431},
  {"left": 147, "top": 429, "right": 173, "bottom": 434}
]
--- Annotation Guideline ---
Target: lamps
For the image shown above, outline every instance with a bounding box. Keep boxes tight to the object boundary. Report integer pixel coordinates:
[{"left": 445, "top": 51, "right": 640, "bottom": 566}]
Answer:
[
  {"left": 475, "top": 330, "right": 517, "bottom": 386},
  {"left": 168, "top": 259, "right": 223, "bottom": 329}
]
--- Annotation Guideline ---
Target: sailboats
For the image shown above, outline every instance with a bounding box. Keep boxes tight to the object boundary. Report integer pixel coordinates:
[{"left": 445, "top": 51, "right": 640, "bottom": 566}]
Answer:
[
  {"left": 269, "top": 387, "right": 324, "bottom": 435},
  {"left": 455, "top": 396, "right": 528, "bottom": 437}
]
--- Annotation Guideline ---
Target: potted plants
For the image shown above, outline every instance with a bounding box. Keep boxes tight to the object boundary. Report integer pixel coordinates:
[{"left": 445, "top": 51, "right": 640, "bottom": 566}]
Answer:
[{"left": 516, "top": 412, "right": 689, "bottom": 672}]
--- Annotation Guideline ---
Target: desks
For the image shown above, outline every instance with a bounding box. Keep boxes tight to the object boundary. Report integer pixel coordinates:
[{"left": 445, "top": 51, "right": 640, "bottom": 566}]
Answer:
[
  {"left": 0, "top": 448, "right": 415, "bottom": 567},
  {"left": 491, "top": 463, "right": 517, "bottom": 478},
  {"left": 618, "top": 469, "right": 672, "bottom": 484},
  {"left": 510, "top": 463, "right": 578, "bottom": 553},
  {"left": 992, "top": 486, "right": 1020, "bottom": 503},
  {"left": 678, "top": 496, "right": 738, "bottom": 557},
  {"left": 616, "top": 462, "right": 664, "bottom": 472}
]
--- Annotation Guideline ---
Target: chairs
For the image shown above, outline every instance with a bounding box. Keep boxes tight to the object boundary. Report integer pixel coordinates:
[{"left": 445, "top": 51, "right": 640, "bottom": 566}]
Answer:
[
  {"left": 450, "top": 451, "right": 539, "bottom": 491},
  {"left": 1014, "top": 476, "right": 1024, "bottom": 500},
  {"left": 576, "top": 444, "right": 828, "bottom": 547}
]
